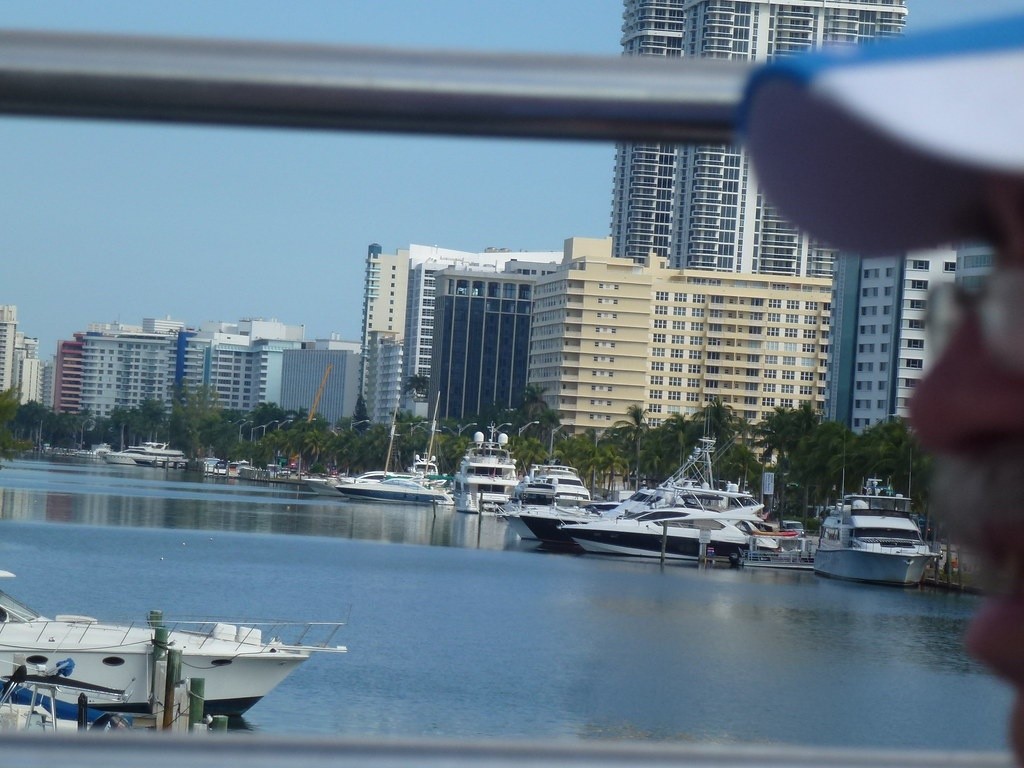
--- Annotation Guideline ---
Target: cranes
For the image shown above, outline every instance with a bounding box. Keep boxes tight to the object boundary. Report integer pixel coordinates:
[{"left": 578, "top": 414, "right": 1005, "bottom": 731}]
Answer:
[{"left": 305, "top": 363, "right": 336, "bottom": 424}]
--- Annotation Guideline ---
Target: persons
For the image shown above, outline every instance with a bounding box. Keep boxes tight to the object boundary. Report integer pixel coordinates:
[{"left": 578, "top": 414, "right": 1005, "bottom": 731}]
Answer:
[{"left": 742, "top": 13, "right": 1023, "bottom": 768}]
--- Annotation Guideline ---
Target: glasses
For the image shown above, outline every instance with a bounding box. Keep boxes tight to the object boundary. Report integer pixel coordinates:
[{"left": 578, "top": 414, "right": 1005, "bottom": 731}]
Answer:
[{"left": 926, "top": 272, "right": 1024, "bottom": 377}]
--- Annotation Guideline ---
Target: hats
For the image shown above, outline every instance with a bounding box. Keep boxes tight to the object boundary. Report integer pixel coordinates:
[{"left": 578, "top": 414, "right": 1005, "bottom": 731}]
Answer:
[{"left": 741, "top": 13, "right": 1022, "bottom": 255}]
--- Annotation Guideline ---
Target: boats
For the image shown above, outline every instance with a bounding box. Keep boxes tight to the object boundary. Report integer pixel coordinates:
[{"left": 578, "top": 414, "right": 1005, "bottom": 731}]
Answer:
[
  {"left": 0, "top": 570, "right": 359, "bottom": 735},
  {"left": 812, "top": 481, "right": 945, "bottom": 590},
  {"left": 105, "top": 441, "right": 804, "bottom": 566}
]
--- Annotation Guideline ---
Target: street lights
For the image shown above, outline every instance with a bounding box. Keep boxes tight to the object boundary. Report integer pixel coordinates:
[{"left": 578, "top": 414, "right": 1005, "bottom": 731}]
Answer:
[{"left": 6, "top": 408, "right": 715, "bottom": 493}]
